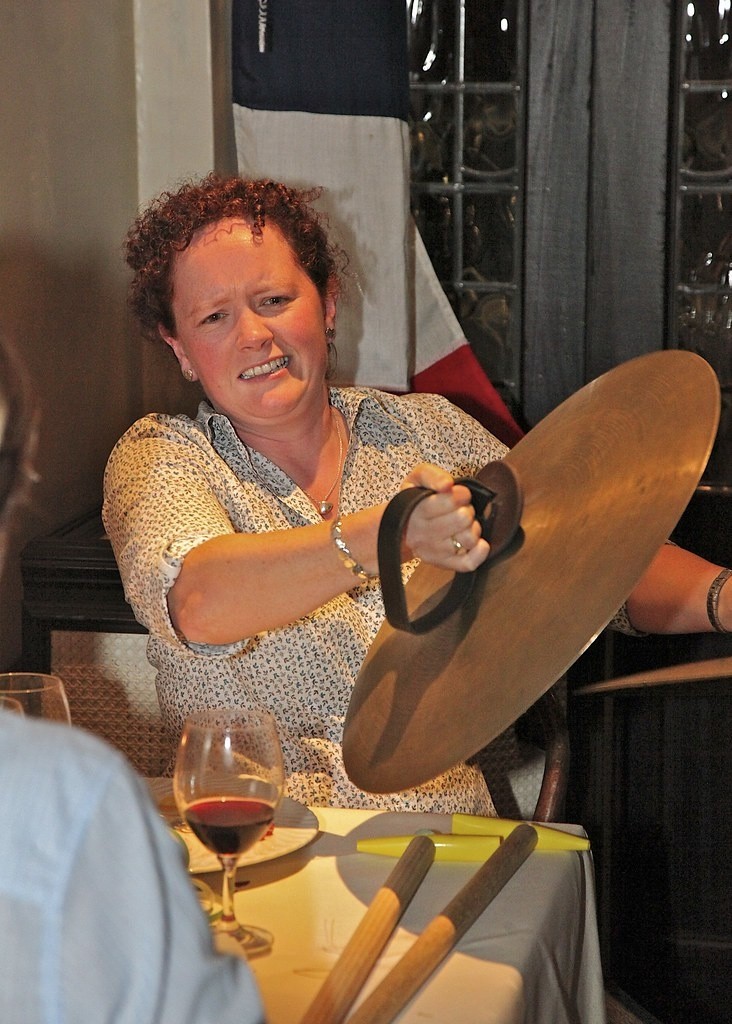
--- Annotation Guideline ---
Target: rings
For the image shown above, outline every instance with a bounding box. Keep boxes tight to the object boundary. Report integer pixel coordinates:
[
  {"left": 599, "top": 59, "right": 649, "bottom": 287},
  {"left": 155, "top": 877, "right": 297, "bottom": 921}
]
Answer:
[{"left": 450, "top": 535, "right": 462, "bottom": 555}]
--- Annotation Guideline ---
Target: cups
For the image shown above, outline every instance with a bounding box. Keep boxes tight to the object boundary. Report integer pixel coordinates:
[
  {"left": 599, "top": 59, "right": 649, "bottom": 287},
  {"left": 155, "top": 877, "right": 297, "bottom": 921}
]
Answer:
[{"left": 0, "top": 673, "right": 71, "bottom": 726}]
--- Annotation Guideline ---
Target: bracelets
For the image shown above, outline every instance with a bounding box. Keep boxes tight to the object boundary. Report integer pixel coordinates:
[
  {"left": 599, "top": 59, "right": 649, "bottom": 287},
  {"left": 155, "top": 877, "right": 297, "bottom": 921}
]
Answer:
[
  {"left": 331, "top": 516, "right": 381, "bottom": 582},
  {"left": 705, "top": 568, "right": 731, "bottom": 634}
]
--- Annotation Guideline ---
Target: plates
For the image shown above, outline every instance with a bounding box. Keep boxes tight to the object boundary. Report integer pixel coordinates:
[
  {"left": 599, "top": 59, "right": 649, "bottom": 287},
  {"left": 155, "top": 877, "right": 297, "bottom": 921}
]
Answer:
[{"left": 144, "top": 778, "right": 318, "bottom": 874}]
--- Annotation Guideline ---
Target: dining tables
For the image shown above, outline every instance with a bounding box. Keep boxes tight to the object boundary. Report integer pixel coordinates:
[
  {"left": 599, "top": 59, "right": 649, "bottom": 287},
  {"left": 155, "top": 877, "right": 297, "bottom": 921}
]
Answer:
[{"left": 188, "top": 807, "right": 586, "bottom": 1023}]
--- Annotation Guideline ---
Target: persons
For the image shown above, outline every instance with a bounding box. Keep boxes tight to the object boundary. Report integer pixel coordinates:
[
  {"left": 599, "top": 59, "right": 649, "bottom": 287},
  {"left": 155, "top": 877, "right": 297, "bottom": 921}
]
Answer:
[
  {"left": 0, "top": 321, "right": 270, "bottom": 1024},
  {"left": 99, "top": 171, "right": 732, "bottom": 820}
]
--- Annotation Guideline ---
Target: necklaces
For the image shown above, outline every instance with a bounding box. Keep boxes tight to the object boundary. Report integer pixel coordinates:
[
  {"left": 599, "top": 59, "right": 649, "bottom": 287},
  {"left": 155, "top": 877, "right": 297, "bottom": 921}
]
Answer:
[{"left": 290, "top": 404, "right": 344, "bottom": 514}]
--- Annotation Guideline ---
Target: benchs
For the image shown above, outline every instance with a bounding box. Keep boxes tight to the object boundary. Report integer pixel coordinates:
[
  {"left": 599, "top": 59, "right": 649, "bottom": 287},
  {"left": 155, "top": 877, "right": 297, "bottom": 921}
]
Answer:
[{"left": 5, "top": 605, "right": 576, "bottom": 829}]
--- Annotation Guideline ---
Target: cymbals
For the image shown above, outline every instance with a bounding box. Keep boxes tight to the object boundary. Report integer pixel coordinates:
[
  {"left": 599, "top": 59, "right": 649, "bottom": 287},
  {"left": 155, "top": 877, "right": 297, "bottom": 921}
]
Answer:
[
  {"left": 341, "top": 351, "right": 721, "bottom": 797},
  {"left": 582, "top": 651, "right": 732, "bottom": 696}
]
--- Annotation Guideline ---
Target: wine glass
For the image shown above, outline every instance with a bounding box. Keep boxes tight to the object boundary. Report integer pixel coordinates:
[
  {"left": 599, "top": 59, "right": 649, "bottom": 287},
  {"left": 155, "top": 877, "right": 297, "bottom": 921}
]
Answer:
[{"left": 173, "top": 710, "right": 284, "bottom": 954}]
